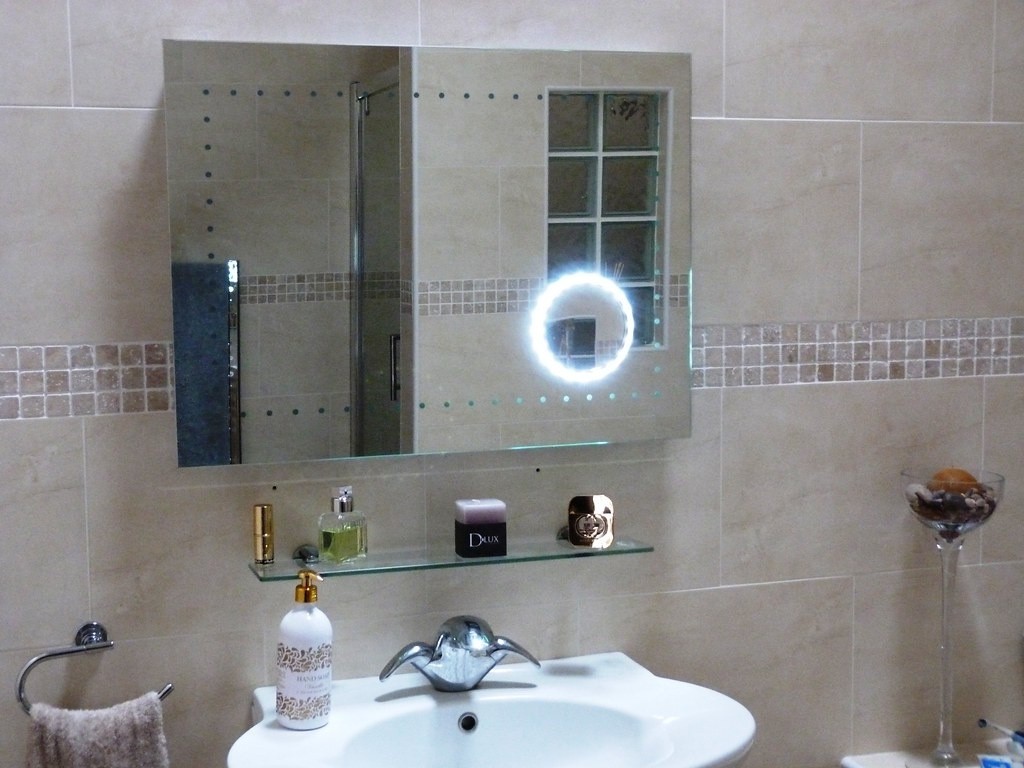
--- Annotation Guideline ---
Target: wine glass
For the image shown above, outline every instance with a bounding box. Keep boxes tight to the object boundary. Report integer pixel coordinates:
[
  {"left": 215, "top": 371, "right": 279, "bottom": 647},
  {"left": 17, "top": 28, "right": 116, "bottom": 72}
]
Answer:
[{"left": 899, "top": 466, "right": 1007, "bottom": 768}]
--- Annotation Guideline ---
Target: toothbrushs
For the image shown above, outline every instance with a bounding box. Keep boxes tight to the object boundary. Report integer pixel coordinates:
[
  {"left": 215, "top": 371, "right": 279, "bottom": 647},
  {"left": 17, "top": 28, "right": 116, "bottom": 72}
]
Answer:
[{"left": 977, "top": 717, "right": 1019, "bottom": 742}]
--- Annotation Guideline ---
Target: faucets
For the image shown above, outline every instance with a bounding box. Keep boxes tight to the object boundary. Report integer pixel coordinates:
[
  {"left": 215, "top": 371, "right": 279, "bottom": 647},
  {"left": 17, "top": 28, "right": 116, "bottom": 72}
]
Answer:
[{"left": 378, "top": 615, "right": 541, "bottom": 691}]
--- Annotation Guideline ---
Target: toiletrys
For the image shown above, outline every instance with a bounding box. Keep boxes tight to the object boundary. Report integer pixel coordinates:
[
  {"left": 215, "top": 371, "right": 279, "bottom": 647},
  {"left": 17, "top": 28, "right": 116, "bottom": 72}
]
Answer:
[
  {"left": 976, "top": 752, "right": 1013, "bottom": 767},
  {"left": 454, "top": 499, "right": 507, "bottom": 558},
  {"left": 275, "top": 569, "right": 331, "bottom": 730}
]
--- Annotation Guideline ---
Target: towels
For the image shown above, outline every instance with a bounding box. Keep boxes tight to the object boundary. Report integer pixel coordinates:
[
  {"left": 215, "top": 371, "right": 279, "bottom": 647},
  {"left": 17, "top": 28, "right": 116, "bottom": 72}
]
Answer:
[{"left": 27, "top": 691, "right": 169, "bottom": 768}]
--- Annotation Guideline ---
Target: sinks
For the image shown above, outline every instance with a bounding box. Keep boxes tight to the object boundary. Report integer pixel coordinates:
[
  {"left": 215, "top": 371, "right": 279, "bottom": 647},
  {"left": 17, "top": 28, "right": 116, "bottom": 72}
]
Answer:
[{"left": 228, "top": 652, "right": 757, "bottom": 768}]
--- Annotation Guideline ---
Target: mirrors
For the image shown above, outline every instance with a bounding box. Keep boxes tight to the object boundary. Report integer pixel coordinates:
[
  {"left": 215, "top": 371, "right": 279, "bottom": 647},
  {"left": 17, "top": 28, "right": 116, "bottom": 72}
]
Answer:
[{"left": 163, "top": 39, "right": 691, "bottom": 468}]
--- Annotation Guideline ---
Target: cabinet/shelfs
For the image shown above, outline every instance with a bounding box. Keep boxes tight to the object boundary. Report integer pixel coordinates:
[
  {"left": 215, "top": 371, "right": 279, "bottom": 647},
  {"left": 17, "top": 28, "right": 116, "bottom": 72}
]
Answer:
[{"left": 542, "top": 86, "right": 673, "bottom": 359}]
